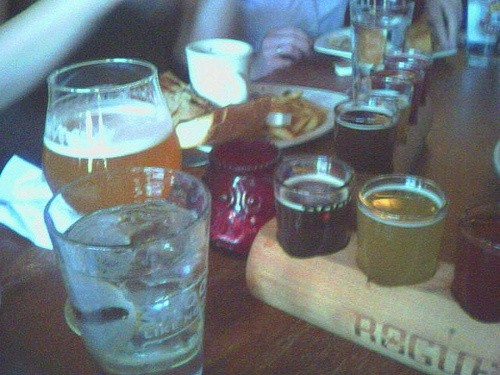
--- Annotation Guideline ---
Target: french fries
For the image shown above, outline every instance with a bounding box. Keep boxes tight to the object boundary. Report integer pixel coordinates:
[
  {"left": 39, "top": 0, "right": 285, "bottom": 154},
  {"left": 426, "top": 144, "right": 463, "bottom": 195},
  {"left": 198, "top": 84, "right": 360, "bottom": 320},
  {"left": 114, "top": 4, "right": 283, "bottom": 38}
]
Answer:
[{"left": 266, "top": 91, "right": 326, "bottom": 142}]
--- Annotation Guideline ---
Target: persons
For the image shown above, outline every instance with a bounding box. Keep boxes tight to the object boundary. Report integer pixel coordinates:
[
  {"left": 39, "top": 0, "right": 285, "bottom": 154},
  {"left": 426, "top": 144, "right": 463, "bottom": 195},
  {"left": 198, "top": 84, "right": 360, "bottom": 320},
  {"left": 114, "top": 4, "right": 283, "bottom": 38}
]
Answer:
[
  {"left": 238, "top": 0, "right": 464, "bottom": 81},
  {"left": 0, "top": 0, "right": 243, "bottom": 145}
]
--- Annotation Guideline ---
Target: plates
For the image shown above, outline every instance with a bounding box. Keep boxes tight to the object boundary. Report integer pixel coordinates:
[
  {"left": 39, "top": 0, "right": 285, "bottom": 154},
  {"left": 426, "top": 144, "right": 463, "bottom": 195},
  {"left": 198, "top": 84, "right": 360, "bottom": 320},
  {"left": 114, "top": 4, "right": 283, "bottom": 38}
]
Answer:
[
  {"left": 160, "top": 81, "right": 349, "bottom": 152},
  {"left": 312, "top": 23, "right": 458, "bottom": 60}
]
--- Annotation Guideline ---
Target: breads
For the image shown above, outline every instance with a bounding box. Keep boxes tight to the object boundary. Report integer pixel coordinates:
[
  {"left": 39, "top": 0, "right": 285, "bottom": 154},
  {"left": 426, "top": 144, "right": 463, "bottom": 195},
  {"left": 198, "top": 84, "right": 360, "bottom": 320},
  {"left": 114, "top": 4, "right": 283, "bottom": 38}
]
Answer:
[
  {"left": 174, "top": 97, "right": 270, "bottom": 150},
  {"left": 349, "top": 16, "right": 433, "bottom": 62}
]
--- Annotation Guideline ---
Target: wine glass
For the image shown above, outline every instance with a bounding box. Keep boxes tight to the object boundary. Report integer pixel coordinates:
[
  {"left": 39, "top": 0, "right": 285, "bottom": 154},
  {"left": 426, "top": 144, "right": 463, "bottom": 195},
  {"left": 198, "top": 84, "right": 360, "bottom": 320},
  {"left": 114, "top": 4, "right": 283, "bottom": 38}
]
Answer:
[{"left": 42, "top": 58, "right": 183, "bottom": 336}]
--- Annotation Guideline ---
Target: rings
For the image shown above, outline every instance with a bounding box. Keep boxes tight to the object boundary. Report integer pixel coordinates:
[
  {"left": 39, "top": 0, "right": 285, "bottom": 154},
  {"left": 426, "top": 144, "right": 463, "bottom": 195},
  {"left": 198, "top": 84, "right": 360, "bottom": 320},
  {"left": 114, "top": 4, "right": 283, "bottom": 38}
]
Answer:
[{"left": 276, "top": 43, "right": 288, "bottom": 58}]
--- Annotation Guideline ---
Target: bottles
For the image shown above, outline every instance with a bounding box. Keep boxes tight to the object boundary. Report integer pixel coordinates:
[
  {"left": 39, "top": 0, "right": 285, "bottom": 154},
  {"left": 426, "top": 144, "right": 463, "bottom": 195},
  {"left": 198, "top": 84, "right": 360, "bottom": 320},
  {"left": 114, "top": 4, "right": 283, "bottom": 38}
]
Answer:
[{"left": 201, "top": 137, "right": 296, "bottom": 256}]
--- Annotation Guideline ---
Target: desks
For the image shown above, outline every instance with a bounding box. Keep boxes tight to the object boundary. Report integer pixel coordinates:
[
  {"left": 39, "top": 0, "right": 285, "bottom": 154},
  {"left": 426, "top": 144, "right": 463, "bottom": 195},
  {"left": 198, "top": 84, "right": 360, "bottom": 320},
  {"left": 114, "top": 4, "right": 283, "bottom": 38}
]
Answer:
[{"left": 0, "top": 53, "right": 500, "bottom": 375}]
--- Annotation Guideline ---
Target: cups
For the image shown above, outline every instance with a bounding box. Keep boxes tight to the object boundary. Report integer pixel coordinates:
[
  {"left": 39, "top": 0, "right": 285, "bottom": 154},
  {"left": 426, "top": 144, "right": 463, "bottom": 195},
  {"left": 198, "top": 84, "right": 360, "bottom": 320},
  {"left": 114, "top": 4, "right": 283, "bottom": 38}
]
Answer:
[
  {"left": 451, "top": 199, "right": 500, "bottom": 322},
  {"left": 331, "top": 0, "right": 434, "bottom": 174},
  {"left": 186, "top": 38, "right": 254, "bottom": 107},
  {"left": 356, "top": 173, "right": 451, "bottom": 288},
  {"left": 42, "top": 165, "right": 213, "bottom": 375},
  {"left": 271, "top": 154, "right": 355, "bottom": 258}
]
